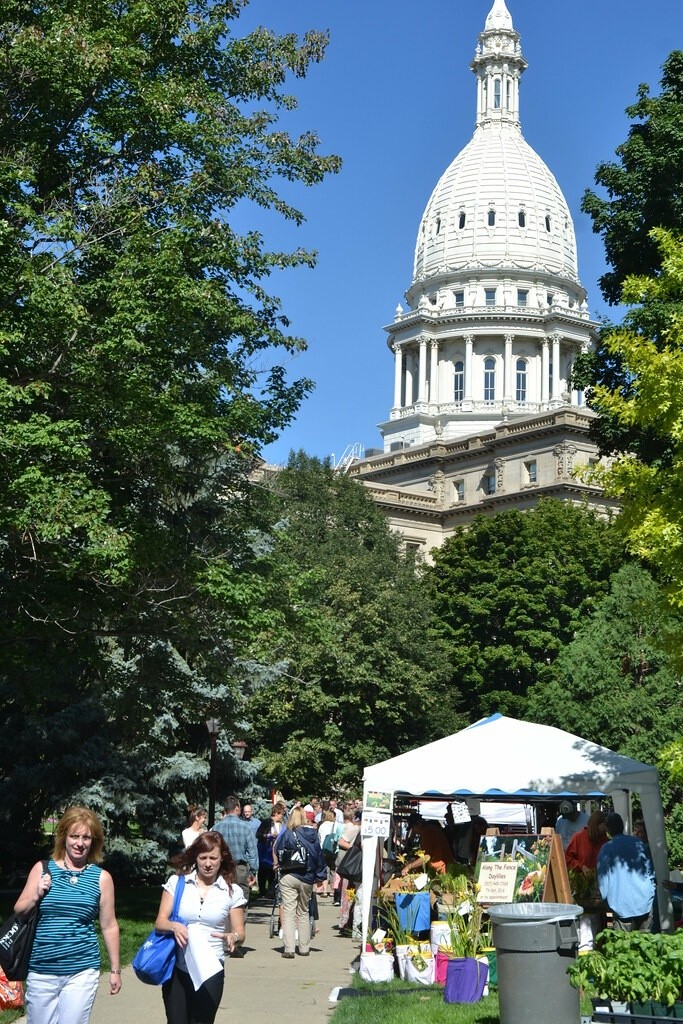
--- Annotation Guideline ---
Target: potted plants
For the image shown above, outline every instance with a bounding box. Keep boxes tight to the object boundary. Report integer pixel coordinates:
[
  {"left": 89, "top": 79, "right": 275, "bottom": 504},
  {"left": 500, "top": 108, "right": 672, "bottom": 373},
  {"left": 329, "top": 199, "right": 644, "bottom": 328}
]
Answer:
[{"left": 565, "top": 930, "right": 683, "bottom": 1023}]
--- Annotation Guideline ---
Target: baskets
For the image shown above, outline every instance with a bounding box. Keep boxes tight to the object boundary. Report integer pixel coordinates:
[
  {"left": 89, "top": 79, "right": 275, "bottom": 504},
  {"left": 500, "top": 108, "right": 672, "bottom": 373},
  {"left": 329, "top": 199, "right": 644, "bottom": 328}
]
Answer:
[{"left": 573, "top": 872, "right": 603, "bottom": 913}]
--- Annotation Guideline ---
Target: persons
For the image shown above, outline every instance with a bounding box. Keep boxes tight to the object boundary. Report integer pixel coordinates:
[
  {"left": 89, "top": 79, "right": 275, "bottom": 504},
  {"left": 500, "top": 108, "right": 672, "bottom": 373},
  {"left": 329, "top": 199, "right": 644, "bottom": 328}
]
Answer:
[
  {"left": 596, "top": 813, "right": 656, "bottom": 931},
  {"left": 556, "top": 800, "right": 590, "bottom": 849},
  {"left": 212, "top": 796, "right": 259, "bottom": 956},
  {"left": 243, "top": 804, "right": 261, "bottom": 832},
  {"left": 258, "top": 803, "right": 285, "bottom": 899},
  {"left": 565, "top": 811, "right": 608, "bottom": 880},
  {"left": 156, "top": 831, "right": 247, "bottom": 1024},
  {"left": 443, "top": 804, "right": 490, "bottom": 862},
  {"left": 271, "top": 807, "right": 327, "bottom": 958},
  {"left": 401, "top": 813, "right": 453, "bottom": 877},
  {"left": 289, "top": 797, "right": 363, "bottom": 928},
  {"left": 633, "top": 820, "right": 649, "bottom": 850},
  {"left": 181, "top": 805, "right": 208, "bottom": 848},
  {"left": 14, "top": 807, "right": 122, "bottom": 1024}
]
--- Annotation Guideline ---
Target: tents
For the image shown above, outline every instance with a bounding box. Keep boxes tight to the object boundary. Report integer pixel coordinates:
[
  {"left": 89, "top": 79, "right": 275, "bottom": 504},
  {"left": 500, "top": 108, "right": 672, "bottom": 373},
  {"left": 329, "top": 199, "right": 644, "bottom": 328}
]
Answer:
[{"left": 362, "top": 713, "right": 673, "bottom": 951}]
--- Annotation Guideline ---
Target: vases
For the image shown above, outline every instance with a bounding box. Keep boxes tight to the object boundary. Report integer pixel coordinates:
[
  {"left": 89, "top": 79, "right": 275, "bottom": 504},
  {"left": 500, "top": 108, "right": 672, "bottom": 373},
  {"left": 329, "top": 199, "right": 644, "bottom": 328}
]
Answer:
[{"left": 360, "top": 891, "right": 496, "bottom": 1003}]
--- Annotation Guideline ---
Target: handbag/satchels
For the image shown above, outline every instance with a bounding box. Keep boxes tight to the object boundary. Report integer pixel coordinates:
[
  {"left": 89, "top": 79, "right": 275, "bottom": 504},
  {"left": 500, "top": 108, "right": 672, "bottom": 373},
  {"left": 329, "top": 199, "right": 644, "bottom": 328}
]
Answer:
[
  {"left": 132, "top": 915, "right": 188, "bottom": 985},
  {"left": 336, "top": 847, "right": 362, "bottom": 882},
  {"left": 323, "top": 833, "right": 336, "bottom": 853},
  {"left": 278, "top": 829, "right": 307, "bottom": 871},
  {"left": 0, "top": 907, "right": 39, "bottom": 981},
  {"left": 260, "top": 837, "right": 273, "bottom": 867}
]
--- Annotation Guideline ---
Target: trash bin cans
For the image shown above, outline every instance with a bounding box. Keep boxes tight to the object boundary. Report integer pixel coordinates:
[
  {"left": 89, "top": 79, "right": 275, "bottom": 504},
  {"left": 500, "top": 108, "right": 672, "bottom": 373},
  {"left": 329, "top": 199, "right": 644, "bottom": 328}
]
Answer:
[{"left": 487, "top": 902, "right": 585, "bottom": 1024}]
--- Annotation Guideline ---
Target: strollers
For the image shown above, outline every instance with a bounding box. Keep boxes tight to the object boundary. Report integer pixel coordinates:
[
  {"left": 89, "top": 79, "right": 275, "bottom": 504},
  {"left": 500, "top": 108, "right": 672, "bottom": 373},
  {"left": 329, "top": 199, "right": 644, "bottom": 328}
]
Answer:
[{"left": 269, "top": 867, "right": 315, "bottom": 939}]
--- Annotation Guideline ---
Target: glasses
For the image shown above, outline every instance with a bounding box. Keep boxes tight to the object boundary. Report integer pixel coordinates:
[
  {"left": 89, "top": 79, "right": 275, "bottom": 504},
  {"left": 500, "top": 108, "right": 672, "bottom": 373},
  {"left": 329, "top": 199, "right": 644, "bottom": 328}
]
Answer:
[{"left": 66, "top": 833, "right": 91, "bottom": 841}]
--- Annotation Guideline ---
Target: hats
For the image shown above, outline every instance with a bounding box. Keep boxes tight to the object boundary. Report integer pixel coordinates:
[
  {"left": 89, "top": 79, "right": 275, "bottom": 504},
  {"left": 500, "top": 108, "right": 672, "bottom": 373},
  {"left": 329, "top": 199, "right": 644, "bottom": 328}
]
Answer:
[
  {"left": 406, "top": 813, "right": 421, "bottom": 831},
  {"left": 559, "top": 800, "right": 575, "bottom": 819}
]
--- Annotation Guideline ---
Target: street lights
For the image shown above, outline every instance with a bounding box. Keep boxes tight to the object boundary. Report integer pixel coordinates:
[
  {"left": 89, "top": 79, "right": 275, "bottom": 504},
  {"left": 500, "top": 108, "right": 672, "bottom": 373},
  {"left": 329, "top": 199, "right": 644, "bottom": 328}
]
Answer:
[{"left": 204, "top": 705, "right": 221, "bottom": 831}]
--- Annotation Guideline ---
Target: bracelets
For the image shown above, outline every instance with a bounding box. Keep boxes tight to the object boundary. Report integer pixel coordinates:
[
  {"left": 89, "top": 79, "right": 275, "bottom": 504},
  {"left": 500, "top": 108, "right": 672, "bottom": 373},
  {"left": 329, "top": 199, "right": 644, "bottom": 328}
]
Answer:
[
  {"left": 111, "top": 969, "right": 121, "bottom": 974},
  {"left": 234, "top": 932, "right": 240, "bottom": 943}
]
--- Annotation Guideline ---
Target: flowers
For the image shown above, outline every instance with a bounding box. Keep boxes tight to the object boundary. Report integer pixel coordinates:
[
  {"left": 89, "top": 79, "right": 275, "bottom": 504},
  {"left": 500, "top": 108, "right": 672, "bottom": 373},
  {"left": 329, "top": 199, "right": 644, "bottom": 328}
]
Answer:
[{"left": 346, "top": 850, "right": 494, "bottom": 959}]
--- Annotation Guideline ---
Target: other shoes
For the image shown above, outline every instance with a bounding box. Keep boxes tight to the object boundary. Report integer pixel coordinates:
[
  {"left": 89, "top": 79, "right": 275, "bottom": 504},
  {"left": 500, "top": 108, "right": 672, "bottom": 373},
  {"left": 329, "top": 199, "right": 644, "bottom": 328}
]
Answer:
[
  {"left": 282, "top": 952, "right": 294, "bottom": 958},
  {"left": 232, "top": 946, "right": 243, "bottom": 957},
  {"left": 334, "top": 901, "right": 340, "bottom": 906},
  {"left": 298, "top": 952, "right": 309, "bottom": 956}
]
